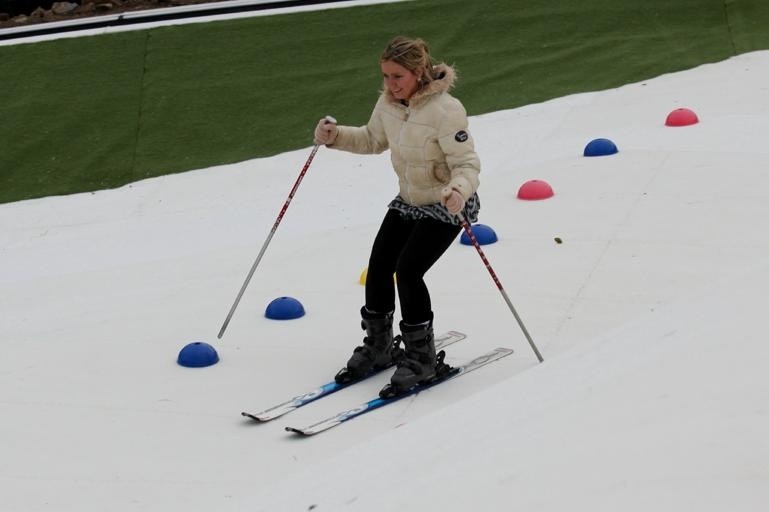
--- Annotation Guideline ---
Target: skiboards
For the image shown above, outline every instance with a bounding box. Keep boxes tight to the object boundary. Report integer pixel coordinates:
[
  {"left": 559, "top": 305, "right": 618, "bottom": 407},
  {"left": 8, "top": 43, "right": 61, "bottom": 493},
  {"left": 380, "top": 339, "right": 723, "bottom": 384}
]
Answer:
[{"left": 241, "top": 330, "right": 514, "bottom": 436}]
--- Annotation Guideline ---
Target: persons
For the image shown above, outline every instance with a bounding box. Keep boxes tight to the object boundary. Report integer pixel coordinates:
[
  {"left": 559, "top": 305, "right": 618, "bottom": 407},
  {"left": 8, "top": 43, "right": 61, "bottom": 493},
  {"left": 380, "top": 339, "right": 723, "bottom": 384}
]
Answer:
[{"left": 312, "top": 33, "right": 483, "bottom": 392}]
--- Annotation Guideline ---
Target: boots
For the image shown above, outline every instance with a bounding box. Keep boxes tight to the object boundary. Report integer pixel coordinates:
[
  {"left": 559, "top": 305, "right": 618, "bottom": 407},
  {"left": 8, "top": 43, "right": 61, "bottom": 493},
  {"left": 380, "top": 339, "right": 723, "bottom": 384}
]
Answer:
[
  {"left": 347, "top": 304, "right": 396, "bottom": 374},
  {"left": 390, "top": 310, "right": 437, "bottom": 387}
]
